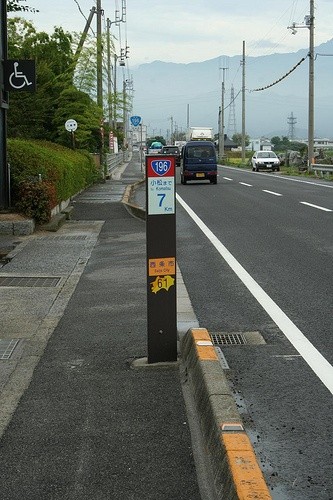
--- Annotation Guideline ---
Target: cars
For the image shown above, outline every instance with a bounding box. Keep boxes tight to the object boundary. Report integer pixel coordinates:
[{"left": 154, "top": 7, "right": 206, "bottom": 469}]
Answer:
[
  {"left": 251, "top": 151, "right": 280, "bottom": 171},
  {"left": 144, "top": 142, "right": 163, "bottom": 154}
]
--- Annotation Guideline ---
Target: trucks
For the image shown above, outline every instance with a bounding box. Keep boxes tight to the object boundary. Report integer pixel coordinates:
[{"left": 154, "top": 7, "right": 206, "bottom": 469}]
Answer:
[{"left": 174, "top": 141, "right": 187, "bottom": 158}]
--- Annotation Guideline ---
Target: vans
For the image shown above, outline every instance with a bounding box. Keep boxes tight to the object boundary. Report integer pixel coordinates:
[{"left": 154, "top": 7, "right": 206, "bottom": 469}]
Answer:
[
  {"left": 161, "top": 146, "right": 180, "bottom": 167},
  {"left": 180, "top": 141, "right": 217, "bottom": 184}
]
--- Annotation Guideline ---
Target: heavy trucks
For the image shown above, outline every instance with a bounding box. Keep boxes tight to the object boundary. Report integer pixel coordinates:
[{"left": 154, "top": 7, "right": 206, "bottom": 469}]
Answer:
[{"left": 190, "top": 127, "right": 213, "bottom": 141}]
